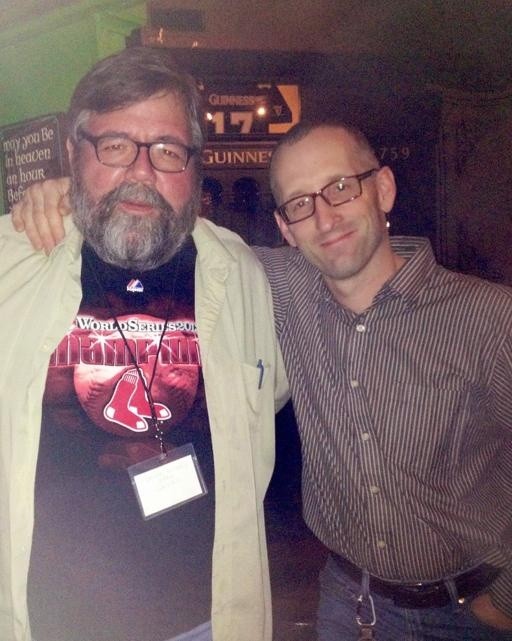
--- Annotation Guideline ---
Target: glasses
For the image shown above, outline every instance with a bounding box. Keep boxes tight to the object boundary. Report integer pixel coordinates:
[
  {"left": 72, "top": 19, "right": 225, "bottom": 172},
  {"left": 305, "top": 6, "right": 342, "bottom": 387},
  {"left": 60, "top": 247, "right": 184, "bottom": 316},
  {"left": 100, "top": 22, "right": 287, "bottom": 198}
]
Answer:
[
  {"left": 276, "top": 169, "right": 376, "bottom": 225},
  {"left": 78, "top": 130, "right": 197, "bottom": 174}
]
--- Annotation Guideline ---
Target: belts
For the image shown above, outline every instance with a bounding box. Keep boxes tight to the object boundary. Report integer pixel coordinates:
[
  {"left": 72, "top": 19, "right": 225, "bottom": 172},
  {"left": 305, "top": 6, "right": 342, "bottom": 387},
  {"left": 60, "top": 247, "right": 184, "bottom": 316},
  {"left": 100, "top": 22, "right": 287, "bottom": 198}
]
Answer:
[{"left": 327, "top": 547, "right": 499, "bottom": 610}]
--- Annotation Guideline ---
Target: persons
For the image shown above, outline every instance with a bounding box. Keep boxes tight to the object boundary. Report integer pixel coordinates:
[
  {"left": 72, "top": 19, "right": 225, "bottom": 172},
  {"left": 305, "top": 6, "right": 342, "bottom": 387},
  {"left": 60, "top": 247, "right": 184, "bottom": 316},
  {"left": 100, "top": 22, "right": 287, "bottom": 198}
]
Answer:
[
  {"left": 202, "top": 172, "right": 233, "bottom": 229},
  {"left": 0, "top": 47, "right": 292, "bottom": 641},
  {"left": 9, "top": 114, "right": 511, "bottom": 641},
  {"left": 229, "top": 177, "right": 282, "bottom": 248}
]
